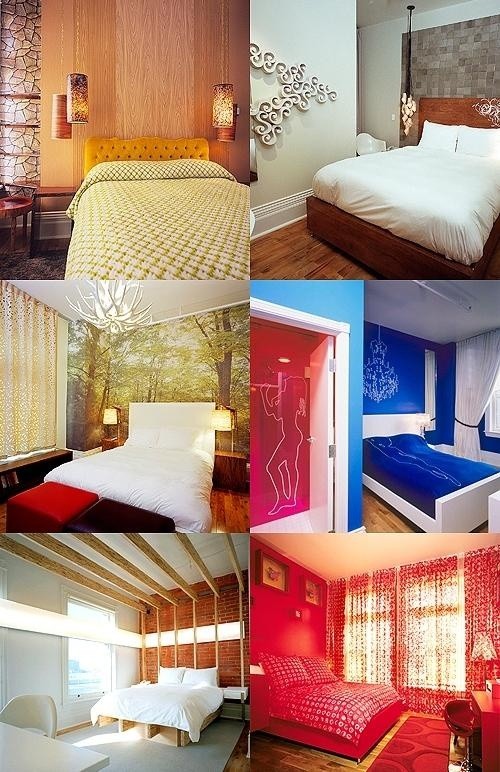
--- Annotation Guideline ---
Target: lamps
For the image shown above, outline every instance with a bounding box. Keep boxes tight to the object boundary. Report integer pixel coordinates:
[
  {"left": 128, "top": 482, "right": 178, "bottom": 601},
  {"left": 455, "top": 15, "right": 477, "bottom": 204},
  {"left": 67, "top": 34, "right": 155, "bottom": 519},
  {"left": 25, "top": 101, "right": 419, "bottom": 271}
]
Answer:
[
  {"left": 62, "top": 281, "right": 154, "bottom": 334},
  {"left": 102, "top": 408, "right": 119, "bottom": 438},
  {"left": 414, "top": 413, "right": 432, "bottom": 436},
  {"left": 211, "top": 406, "right": 235, "bottom": 453},
  {"left": 49, "top": 71, "right": 88, "bottom": 141},
  {"left": 469, "top": 629, "right": 497, "bottom": 688},
  {"left": 400, "top": 0, "right": 417, "bottom": 139}
]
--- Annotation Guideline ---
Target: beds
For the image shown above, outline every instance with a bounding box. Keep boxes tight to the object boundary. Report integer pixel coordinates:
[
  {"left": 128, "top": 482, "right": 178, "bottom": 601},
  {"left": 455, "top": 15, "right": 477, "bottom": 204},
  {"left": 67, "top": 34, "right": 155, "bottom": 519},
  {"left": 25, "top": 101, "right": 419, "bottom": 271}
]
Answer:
[
  {"left": 305, "top": 94, "right": 500, "bottom": 280},
  {"left": 363, "top": 412, "right": 500, "bottom": 533},
  {"left": 42, "top": 399, "right": 219, "bottom": 533},
  {"left": 254, "top": 648, "right": 403, "bottom": 762},
  {"left": 90, "top": 663, "right": 226, "bottom": 747},
  {"left": 66, "top": 135, "right": 251, "bottom": 281}
]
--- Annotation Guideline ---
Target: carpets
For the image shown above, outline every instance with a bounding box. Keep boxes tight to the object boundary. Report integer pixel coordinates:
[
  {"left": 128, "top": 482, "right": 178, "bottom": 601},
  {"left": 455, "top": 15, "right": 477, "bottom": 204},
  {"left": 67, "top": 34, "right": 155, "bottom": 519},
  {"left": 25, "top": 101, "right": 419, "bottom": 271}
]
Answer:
[
  {"left": 0, "top": 246, "right": 66, "bottom": 280},
  {"left": 56, "top": 715, "right": 246, "bottom": 771},
  {"left": 362, "top": 715, "right": 451, "bottom": 771}
]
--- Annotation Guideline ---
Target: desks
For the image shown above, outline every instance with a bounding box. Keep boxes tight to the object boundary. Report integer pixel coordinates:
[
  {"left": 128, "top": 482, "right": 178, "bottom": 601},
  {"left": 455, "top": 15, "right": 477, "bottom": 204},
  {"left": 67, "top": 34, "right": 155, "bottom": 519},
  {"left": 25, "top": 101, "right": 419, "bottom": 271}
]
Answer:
[
  {"left": 0, "top": 446, "right": 75, "bottom": 499},
  {"left": 471, "top": 689, "right": 500, "bottom": 772},
  {"left": 487, "top": 490, "right": 500, "bottom": 533},
  {"left": 0, "top": 722, "right": 110, "bottom": 772}
]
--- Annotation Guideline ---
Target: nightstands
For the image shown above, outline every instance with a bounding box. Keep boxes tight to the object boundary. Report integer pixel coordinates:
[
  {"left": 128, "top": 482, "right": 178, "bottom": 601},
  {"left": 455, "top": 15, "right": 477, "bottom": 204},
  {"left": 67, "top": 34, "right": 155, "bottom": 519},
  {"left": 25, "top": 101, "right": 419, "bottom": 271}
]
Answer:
[
  {"left": 222, "top": 684, "right": 248, "bottom": 726},
  {"left": 28, "top": 185, "right": 80, "bottom": 257},
  {"left": 214, "top": 450, "right": 248, "bottom": 490},
  {"left": 101, "top": 436, "right": 125, "bottom": 452},
  {"left": 250, "top": 673, "right": 272, "bottom": 733}
]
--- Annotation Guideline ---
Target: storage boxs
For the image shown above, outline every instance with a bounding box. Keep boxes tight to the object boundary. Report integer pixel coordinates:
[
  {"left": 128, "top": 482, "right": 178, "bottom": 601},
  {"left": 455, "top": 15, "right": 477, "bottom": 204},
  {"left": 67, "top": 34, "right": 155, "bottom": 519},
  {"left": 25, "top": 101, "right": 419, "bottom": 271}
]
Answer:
[{"left": 484, "top": 676, "right": 500, "bottom": 699}]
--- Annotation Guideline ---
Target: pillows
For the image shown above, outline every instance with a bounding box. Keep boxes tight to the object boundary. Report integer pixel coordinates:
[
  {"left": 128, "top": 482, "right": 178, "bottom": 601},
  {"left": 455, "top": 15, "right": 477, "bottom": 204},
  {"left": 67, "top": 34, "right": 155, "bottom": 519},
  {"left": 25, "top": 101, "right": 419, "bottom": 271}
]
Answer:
[
  {"left": 298, "top": 655, "right": 340, "bottom": 684},
  {"left": 418, "top": 118, "right": 459, "bottom": 152},
  {"left": 123, "top": 427, "right": 160, "bottom": 448},
  {"left": 364, "top": 433, "right": 434, "bottom": 459},
  {"left": 182, "top": 667, "right": 219, "bottom": 687},
  {"left": 157, "top": 665, "right": 186, "bottom": 681},
  {"left": 159, "top": 426, "right": 215, "bottom": 452},
  {"left": 456, "top": 123, "right": 500, "bottom": 157},
  {"left": 256, "top": 649, "right": 313, "bottom": 690}
]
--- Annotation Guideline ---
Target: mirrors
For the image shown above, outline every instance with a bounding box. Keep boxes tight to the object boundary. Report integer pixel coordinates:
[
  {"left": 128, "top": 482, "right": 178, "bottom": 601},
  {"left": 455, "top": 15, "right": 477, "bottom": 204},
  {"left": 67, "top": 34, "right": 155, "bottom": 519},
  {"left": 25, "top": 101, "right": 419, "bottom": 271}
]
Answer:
[{"left": 250, "top": 139, "right": 259, "bottom": 183}]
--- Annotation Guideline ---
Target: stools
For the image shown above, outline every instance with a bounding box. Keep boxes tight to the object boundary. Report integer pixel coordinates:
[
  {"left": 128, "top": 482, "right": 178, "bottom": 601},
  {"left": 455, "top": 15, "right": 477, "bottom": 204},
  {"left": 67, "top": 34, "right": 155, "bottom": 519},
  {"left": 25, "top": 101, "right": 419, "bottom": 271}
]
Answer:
[
  {"left": 5, "top": 480, "right": 99, "bottom": 532},
  {"left": 65, "top": 497, "right": 176, "bottom": 533}
]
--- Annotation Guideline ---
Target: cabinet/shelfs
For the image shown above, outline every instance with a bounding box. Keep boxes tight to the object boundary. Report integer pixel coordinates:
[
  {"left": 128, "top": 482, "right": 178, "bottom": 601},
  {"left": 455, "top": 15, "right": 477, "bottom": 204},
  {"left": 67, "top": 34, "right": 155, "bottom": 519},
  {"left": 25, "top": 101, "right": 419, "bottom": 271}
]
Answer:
[{"left": 0, "top": 93, "right": 40, "bottom": 191}]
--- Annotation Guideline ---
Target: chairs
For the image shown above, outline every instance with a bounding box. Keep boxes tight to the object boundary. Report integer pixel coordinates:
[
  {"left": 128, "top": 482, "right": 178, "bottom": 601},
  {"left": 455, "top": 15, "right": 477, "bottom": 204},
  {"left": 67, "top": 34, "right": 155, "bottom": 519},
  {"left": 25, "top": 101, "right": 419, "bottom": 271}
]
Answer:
[
  {"left": 1, "top": 179, "right": 38, "bottom": 253},
  {"left": 442, "top": 699, "right": 482, "bottom": 772},
  {"left": 0, "top": 691, "right": 58, "bottom": 741},
  {"left": 354, "top": 131, "right": 388, "bottom": 156}
]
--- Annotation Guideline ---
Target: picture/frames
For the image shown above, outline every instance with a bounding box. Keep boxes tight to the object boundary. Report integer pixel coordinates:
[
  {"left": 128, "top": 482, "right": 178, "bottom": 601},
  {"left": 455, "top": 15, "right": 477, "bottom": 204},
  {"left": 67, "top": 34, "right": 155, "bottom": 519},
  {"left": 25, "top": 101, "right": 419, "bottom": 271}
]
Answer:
[
  {"left": 254, "top": 549, "right": 291, "bottom": 593},
  {"left": 299, "top": 574, "right": 323, "bottom": 607}
]
